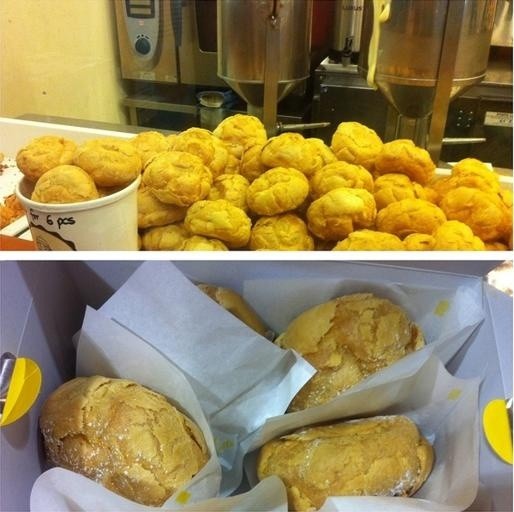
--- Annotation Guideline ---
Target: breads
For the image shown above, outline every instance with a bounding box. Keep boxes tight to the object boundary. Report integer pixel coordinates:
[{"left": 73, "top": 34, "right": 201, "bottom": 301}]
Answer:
[
  {"left": 137, "top": 186, "right": 186, "bottom": 228},
  {"left": 143, "top": 225, "right": 186, "bottom": 251},
  {"left": 184, "top": 201, "right": 251, "bottom": 248},
  {"left": 210, "top": 175, "right": 249, "bottom": 212},
  {"left": 224, "top": 154, "right": 240, "bottom": 174},
  {"left": 404, "top": 234, "right": 433, "bottom": 250},
  {"left": 331, "top": 230, "right": 406, "bottom": 251},
  {"left": 376, "top": 139, "right": 435, "bottom": 184},
  {"left": 260, "top": 133, "right": 312, "bottom": 174},
  {"left": 16, "top": 135, "right": 75, "bottom": 179},
  {"left": 240, "top": 146, "right": 267, "bottom": 180},
  {"left": 249, "top": 214, "right": 314, "bottom": 251},
  {"left": 306, "top": 188, "right": 376, "bottom": 240},
  {"left": 375, "top": 199, "right": 446, "bottom": 240},
  {"left": 453, "top": 159, "right": 499, "bottom": 191},
  {"left": 72, "top": 137, "right": 140, "bottom": 185},
  {"left": 214, "top": 114, "right": 267, "bottom": 157},
  {"left": 506, "top": 202, "right": 513, "bottom": 249},
  {"left": 433, "top": 176, "right": 450, "bottom": 194},
  {"left": 30, "top": 164, "right": 98, "bottom": 204},
  {"left": 497, "top": 189, "right": 512, "bottom": 207},
  {"left": 257, "top": 415, "right": 434, "bottom": 508},
  {"left": 375, "top": 174, "right": 438, "bottom": 212},
  {"left": 307, "top": 138, "right": 336, "bottom": 170},
  {"left": 38, "top": 375, "right": 209, "bottom": 507},
  {"left": 310, "top": 162, "right": 374, "bottom": 200},
  {"left": 172, "top": 128, "right": 226, "bottom": 176},
  {"left": 486, "top": 243, "right": 508, "bottom": 251},
  {"left": 441, "top": 188, "right": 505, "bottom": 240},
  {"left": 433, "top": 220, "right": 484, "bottom": 251},
  {"left": 175, "top": 236, "right": 229, "bottom": 251},
  {"left": 143, "top": 151, "right": 212, "bottom": 207},
  {"left": 197, "top": 285, "right": 275, "bottom": 341},
  {"left": 138, "top": 235, "right": 142, "bottom": 249},
  {"left": 275, "top": 292, "right": 425, "bottom": 413},
  {"left": 246, "top": 167, "right": 309, "bottom": 216},
  {"left": 331, "top": 122, "right": 384, "bottom": 170},
  {"left": 133, "top": 131, "right": 169, "bottom": 171}
]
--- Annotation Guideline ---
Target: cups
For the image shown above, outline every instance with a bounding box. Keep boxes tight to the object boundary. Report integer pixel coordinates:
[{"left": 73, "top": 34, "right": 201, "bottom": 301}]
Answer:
[{"left": 13, "top": 171, "right": 143, "bottom": 261}]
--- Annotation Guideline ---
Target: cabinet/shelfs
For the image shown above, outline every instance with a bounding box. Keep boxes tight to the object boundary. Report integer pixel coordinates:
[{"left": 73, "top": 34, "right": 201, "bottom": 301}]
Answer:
[{"left": 314, "top": 50, "right": 513, "bottom": 168}]
